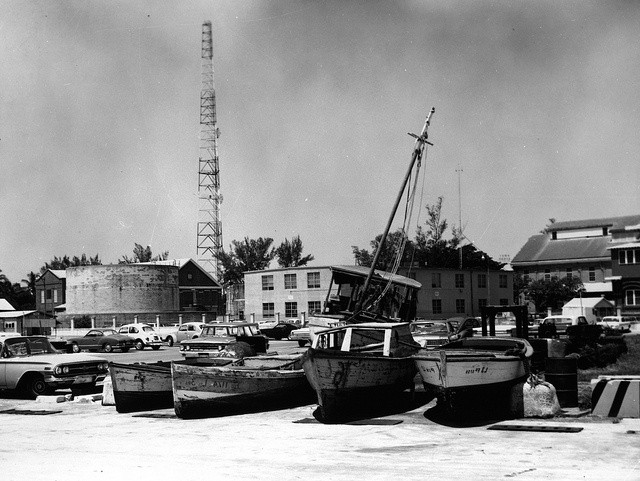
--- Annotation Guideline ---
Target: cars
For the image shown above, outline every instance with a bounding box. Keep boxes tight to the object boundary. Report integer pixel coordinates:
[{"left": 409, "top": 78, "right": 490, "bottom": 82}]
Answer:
[
  {"left": 409, "top": 320, "right": 459, "bottom": 351},
  {"left": 445, "top": 316, "right": 480, "bottom": 335},
  {"left": 116, "top": 323, "right": 164, "bottom": 351},
  {"left": 473, "top": 318, "right": 516, "bottom": 337},
  {"left": 596, "top": 314, "right": 633, "bottom": 334},
  {"left": 180, "top": 322, "right": 269, "bottom": 360},
  {"left": 0, "top": 335, "right": 109, "bottom": 398},
  {"left": 162, "top": 321, "right": 213, "bottom": 348},
  {"left": 59, "top": 328, "right": 135, "bottom": 354},
  {"left": 260, "top": 324, "right": 298, "bottom": 341},
  {"left": 290, "top": 320, "right": 314, "bottom": 348}
]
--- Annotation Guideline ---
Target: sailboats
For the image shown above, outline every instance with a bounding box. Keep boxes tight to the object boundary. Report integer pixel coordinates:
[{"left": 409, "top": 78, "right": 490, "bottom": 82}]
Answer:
[{"left": 305, "top": 106, "right": 437, "bottom": 357}]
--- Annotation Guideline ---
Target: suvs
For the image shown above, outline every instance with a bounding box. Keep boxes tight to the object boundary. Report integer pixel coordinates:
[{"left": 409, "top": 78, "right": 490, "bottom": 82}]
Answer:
[{"left": 537, "top": 315, "right": 589, "bottom": 343}]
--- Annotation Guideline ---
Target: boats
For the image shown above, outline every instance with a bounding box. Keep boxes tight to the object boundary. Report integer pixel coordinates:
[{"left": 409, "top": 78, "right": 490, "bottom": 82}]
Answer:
[
  {"left": 301, "top": 322, "right": 420, "bottom": 424},
  {"left": 413, "top": 335, "right": 536, "bottom": 422},
  {"left": 169, "top": 355, "right": 317, "bottom": 421},
  {"left": 108, "top": 359, "right": 176, "bottom": 413}
]
